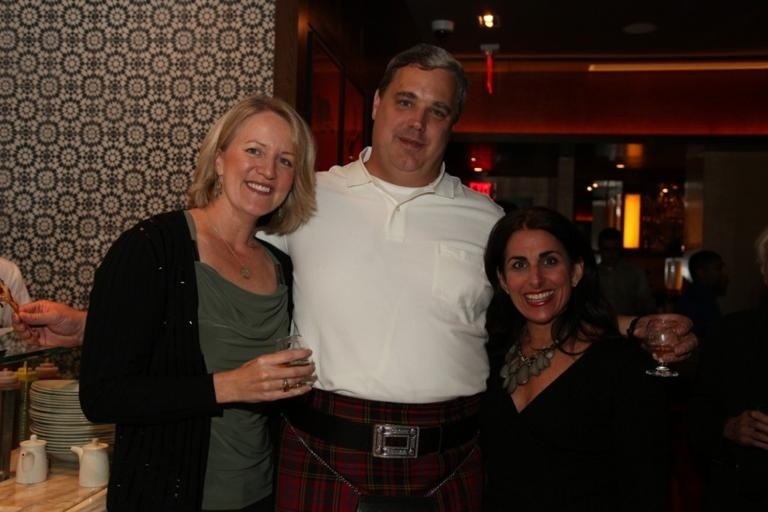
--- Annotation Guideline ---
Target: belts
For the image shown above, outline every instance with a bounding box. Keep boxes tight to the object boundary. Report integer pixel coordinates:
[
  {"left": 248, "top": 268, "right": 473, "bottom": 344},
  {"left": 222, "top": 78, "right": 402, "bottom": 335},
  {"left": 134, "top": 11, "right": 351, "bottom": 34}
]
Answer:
[{"left": 287, "top": 407, "right": 478, "bottom": 459}]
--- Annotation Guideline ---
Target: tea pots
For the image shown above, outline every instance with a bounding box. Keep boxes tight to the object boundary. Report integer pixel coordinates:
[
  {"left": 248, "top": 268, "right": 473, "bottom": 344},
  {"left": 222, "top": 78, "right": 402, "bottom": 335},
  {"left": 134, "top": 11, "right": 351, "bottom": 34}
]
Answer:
[
  {"left": 17, "top": 433, "right": 52, "bottom": 486},
  {"left": 69, "top": 435, "right": 111, "bottom": 488}
]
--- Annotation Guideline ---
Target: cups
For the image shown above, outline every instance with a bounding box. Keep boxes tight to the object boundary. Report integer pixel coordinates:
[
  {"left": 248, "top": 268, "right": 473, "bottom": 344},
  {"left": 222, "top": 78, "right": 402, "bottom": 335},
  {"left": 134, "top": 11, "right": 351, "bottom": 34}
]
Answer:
[{"left": 274, "top": 335, "right": 311, "bottom": 389}]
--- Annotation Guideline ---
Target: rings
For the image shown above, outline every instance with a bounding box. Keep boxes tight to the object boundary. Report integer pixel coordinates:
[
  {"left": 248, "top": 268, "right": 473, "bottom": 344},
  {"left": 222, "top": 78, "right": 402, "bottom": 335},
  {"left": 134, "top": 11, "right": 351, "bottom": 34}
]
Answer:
[{"left": 283, "top": 378, "right": 289, "bottom": 392}]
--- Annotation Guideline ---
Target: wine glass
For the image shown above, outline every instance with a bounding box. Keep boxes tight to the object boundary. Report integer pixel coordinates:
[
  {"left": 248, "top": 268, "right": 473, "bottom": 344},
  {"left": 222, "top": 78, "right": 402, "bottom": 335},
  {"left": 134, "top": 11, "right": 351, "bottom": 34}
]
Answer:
[{"left": 642, "top": 318, "right": 681, "bottom": 377}]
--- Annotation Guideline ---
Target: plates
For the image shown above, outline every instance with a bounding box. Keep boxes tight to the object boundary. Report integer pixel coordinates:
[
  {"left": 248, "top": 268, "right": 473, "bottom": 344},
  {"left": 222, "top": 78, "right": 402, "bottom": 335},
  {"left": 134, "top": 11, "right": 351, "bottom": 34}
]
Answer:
[{"left": 27, "top": 377, "right": 117, "bottom": 465}]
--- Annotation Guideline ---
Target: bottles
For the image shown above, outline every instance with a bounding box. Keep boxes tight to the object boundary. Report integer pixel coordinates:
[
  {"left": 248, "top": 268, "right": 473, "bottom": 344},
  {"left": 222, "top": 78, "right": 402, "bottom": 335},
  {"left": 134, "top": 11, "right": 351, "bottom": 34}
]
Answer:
[
  {"left": 10, "top": 356, "right": 57, "bottom": 452},
  {"left": 0, "top": 366, "right": 20, "bottom": 482}
]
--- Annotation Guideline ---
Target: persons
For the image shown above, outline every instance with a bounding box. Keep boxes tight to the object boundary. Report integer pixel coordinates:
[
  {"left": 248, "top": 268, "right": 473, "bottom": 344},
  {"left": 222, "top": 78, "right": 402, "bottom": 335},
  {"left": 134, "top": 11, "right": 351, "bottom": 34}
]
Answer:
[
  {"left": 79, "top": 95, "right": 316, "bottom": 512},
  {"left": 597, "top": 227, "right": 728, "bottom": 342},
  {"left": 477, "top": 207, "right": 669, "bottom": 512},
  {"left": 687, "top": 227, "right": 768, "bottom": 512},
  {"left": 9, "top": 42, "right": 699, "bottom": 512}
]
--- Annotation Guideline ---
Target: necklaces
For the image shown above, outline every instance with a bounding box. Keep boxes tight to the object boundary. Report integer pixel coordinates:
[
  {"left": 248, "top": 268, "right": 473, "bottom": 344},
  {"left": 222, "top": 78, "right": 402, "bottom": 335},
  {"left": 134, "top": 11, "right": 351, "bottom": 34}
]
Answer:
[
  {"left": 499, "top": 335, "right": 566, "bottom": 394},
  {"left": 212, "top": 227, "right": 250, "bottom": 279}
]
